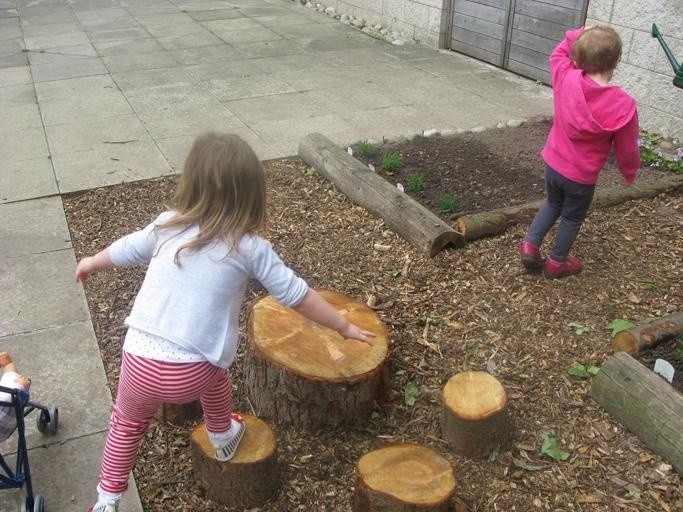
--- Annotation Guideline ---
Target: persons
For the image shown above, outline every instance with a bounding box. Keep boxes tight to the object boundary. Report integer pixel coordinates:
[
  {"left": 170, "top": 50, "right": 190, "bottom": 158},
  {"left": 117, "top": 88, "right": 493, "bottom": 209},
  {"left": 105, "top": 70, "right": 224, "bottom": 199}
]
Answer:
[
  {"left": 72, "top": 129, "right": 379, "bottom": 512},
  {"left": 516, "top": 23, "right": 642, "bottom": 282},
  {"left": 0, "top": 351, "right": 32, "bottom": 404}
]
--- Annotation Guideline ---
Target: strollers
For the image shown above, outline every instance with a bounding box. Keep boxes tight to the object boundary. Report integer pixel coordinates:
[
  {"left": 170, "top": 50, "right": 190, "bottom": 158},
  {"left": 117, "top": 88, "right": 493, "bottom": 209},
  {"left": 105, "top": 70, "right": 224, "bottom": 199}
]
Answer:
[{"left": 0, "top": 386, "right": 59, "bottom": 512}]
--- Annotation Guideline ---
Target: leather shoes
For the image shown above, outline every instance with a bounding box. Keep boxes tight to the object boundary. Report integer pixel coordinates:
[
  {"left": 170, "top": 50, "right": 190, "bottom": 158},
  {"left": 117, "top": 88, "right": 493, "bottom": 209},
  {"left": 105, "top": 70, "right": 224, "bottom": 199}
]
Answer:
[
  {"left": 543, "top": 254, "right": 585, "bottom": 279},
  {"left": 519, "top": 238, "right": 547, "bottom": 272}
]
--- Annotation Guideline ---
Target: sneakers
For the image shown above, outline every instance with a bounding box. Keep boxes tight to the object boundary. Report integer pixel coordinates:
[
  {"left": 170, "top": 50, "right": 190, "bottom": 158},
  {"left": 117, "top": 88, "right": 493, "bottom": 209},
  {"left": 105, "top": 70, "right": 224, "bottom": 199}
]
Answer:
[
  {"left": 86, "top": 500, "right": 118, "bottom": 512},
  {"left": 211, "top": 412, "right": 247, "bottom": 464}
]
